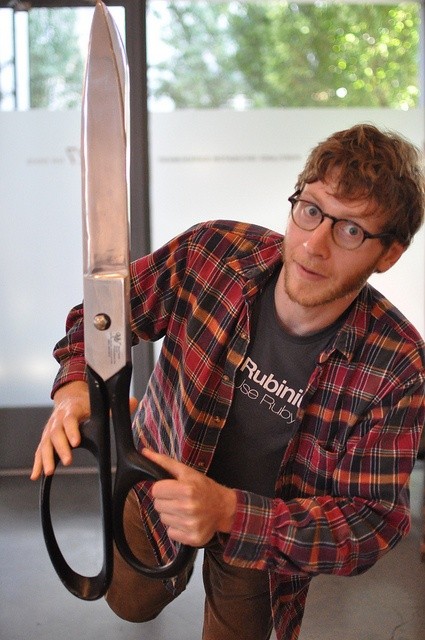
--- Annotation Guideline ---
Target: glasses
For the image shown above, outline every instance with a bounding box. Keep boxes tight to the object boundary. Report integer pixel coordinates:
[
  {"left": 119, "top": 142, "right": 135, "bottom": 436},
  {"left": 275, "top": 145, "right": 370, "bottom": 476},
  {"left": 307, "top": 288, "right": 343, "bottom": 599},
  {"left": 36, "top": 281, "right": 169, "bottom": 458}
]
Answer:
[{"left": 287, "top": 188, "right": 391, "bottom": 249}]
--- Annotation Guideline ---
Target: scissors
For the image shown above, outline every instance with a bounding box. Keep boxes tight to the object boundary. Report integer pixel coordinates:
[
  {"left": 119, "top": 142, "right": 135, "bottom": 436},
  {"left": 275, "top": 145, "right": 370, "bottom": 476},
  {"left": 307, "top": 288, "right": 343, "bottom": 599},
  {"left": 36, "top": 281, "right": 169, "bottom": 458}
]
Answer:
[{"left": 39, "top": 2, "right": 196, "bottom": 601}]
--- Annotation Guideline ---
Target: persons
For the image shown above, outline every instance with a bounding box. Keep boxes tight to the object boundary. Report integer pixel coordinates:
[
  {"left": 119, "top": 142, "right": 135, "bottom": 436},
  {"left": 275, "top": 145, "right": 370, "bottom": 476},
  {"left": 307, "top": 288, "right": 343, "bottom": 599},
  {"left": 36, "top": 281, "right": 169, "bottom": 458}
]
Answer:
[{"left": 28, "top": 121, "right": 425, "bottom": 639}]
